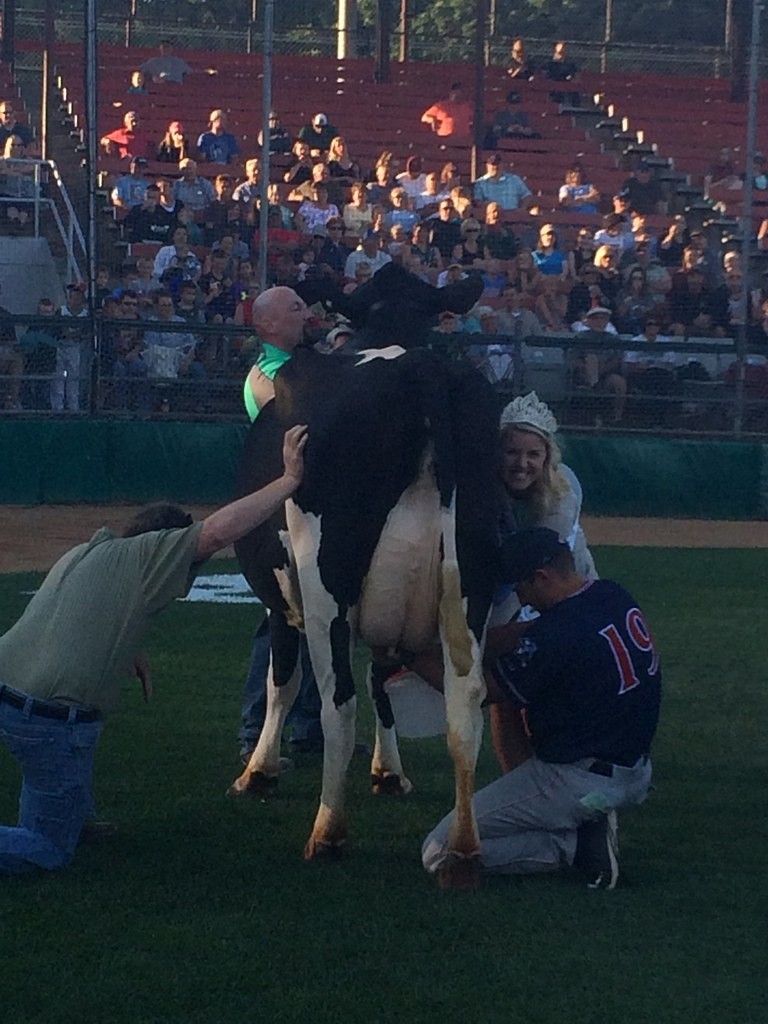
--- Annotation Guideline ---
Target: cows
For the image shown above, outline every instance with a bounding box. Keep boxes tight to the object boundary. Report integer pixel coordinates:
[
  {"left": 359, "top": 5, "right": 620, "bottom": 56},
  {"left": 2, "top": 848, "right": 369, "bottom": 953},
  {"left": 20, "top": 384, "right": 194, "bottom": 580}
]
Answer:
[{"left": 227, "top": 263, "right": 501, "bottom": 863}]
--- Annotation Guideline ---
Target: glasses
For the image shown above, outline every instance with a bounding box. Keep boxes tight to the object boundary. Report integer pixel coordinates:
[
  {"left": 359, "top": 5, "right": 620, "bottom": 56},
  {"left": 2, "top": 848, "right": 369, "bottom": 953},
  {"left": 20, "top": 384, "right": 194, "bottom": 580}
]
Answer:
[
  {"left": 646, "top": 322, "right": 660, "bottom": 326},
  {"left": 728, "top": 275, "right": 742, "bottom": 279},
  {"left": 1, "top": 111, "right": 14, "bottom": 114},
  {"left": 161, "top": 303, "right": 173, "bottom": 307},
  {"left": 270, "top": 116, "right": 281, "bottom": 121},
  {"left": 329, "top": 226, "right": 343, "bottom": 231},
  {"left": 11, "top": 143, "right": 23, "bottom": 147},
  {"left": 123, "top": 301, "right": 138, "bottom": 307}
]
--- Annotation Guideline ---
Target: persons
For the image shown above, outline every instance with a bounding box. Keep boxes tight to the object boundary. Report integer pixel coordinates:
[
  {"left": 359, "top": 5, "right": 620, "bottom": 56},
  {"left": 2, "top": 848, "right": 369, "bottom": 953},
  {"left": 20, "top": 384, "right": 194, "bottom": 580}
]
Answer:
[
  {"left": 97, "top": 111, "right": 154, "bottom": 161},
  {"left": 126, "top": 71, "right": 149, "bottom": 94},
  {"left": 256, "top": 111, "right": 292, "bottom": 156},
  {"left": 299, "top": 113, "right": 340, "bottom": 158},
  {"left": 421, "top": 82, "right": 475, "bottom": 135},
  {"left": 0, "top": 134, "right": 35, "bottom": 235},
  {"left": 194, "top": 110, "right": 240, "bottom": 164},
  {"left": 156, "top": 121, "right": 189, "bottom": 163},
  {"left": 0, "top": 100, "right": 37, "bottom": 152},
  {"left": 506, "top": 38, "right": 535, "bottom": 82},
  {"left": 1, "top": 291, "right": 91, "bottom": 418},
  {"left": 484, "top": 387, "right": 597, "bottom": 771},
  {"left": 369, "top": 526, "right": 665, "bottom": 889},
  {"left": 539, "top": 42, "right": 583, "bottom": 82},
  {"left": 242, "top": 286, "right": 368, "bottom": 774},
  {"left": 491, "top": 91, "right": 541, "bottom": 139},
  {"left": 0, "top": 422, "right": 309, "bottom": 871},
  {"left": 95, "top": 136, "right": 768, "bottom": 427},
  {"left": 140, "top": 41, "right": 218, "bottom": 84}
]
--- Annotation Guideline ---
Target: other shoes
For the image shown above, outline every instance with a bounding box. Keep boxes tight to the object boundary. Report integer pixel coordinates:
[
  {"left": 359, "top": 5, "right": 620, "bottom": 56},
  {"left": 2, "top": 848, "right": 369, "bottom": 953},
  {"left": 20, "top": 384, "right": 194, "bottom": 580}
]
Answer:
[
  {"left": 288, "top": 733, "right": 370, "bottom": 758},
  {"left": 581, "top": 811, "right": 624, "bottom": 892},
  {"left": 83, "top": 819, "right": 117, "bottom": 838},
  {"left": 240, "top": 738, "right": 294, "bottom": 771}
]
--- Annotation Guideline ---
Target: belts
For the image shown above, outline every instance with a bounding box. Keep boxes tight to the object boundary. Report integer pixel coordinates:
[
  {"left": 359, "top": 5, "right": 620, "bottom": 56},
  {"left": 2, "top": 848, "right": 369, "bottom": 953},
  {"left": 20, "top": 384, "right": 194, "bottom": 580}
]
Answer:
[
  {"left": 574, "top": 757, "right": 613, "bottom": 778},
  {"left": 0, "top": 689, "right": 99, "bottom": 723}
]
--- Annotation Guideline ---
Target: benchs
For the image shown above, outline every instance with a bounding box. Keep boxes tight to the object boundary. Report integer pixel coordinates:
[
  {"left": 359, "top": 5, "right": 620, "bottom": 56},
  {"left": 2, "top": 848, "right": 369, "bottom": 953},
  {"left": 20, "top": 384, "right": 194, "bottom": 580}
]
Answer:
[{"left": 0, "top": 40, "right": 768, "bottom": 259}]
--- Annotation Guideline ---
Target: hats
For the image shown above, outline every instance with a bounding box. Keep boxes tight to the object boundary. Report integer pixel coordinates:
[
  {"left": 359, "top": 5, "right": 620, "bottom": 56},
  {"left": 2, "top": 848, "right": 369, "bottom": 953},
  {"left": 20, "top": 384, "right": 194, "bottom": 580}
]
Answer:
[
  {"left": 65, "top": 282, "right": 87, "bottom": 291},
  {"left": 487, "top": 150, "right": 504, "bottom": 165},
  {"left": 493, "top": 529, "right": 569, "bottom": 577},
  {"left": 586, "top": 307, "right": 613, "bottom": 315},
  {"left": 540, "top": 223, "right": 557, "bottom": 236},
  {"left": 448, "top": 262, "right": 463, "bottom": 271},
  {"left": 315, "top": 114, "right": 328, "bottom": 125},
  {"left": 612, "top": 189, "right": 632, "bottom": 200}
]
